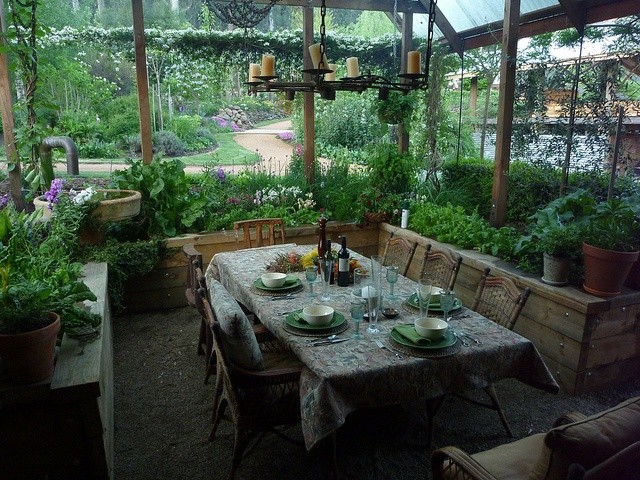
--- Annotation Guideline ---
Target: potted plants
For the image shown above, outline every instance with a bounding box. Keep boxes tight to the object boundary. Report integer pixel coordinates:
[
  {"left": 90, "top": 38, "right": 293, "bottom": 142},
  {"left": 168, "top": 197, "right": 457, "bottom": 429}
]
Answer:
[
  {"left": 0, "top": 208, "right": 59, "bottom": 380},
  {"left": 535, "top": 226, "right": 582, "bottom": 286},
  {"left": 582, "top": 196, "right": 639, "bottom": 297},
  {"left": 361, "top": 189, "right": 388, "bottom": 223}
]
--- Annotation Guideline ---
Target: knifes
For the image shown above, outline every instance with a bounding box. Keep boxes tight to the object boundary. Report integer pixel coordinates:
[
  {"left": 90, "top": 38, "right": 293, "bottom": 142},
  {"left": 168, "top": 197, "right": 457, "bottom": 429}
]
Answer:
[{"left": 308, "top": 338, "right": 353, "bottom": 347}]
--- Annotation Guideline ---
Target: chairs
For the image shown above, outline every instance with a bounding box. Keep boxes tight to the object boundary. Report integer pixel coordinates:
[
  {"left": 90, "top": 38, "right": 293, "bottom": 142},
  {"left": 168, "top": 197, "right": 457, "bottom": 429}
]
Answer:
[
  {"left": 194, "top": 266, "right": 220, "bottom": 423},
  {"left": 377, "top": 232, "right": 418, "bottom": 272},
  {"left": 423, "top": 268, "right": 531, "bottom": 450},
  {"left": 235, "top": 217, "right": 287, "bottom": 249},
  {"left": 417, "top": 243, "right": 464, "bottom": 292},
  {"left": 180, "top": 243, "right": 207, "bottom": 366},
  {"left": 431, "top": 409, "right": 640, "bottom": 479},
  {"left": 198, "top": 289, "right": 341, "bottom": 476}
]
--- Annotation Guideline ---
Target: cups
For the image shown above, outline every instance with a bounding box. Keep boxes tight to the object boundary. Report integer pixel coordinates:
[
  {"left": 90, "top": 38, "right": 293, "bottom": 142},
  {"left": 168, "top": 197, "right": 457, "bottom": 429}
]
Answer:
[
  {"left": 418, "top": 279, "right": 433, "bottom": 320},
  {"left": 353, "top": 267, "right": 379, "bottom": 304},
  {"left": 351, "top": 287, "right": 378, "bottom": 311},
  {"left": 370, "top": 256, "right": 381, "bottom": 289},
  {"left": 382, "top": 294, "right": 401, "bottom": 319},
  {"left": 318, "top": 259, "right": 333, "bottom": 301}
]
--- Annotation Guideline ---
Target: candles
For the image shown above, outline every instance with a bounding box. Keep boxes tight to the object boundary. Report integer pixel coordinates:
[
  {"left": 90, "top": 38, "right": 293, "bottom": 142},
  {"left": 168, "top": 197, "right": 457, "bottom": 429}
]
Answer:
[
  {"left": 407, "top": 51, "right": 420, "bottom": 73},
  {"left": 248, "top": 63, "right": 260, "bottom": 82},
  {"left": 310, "top": 43, "right": 329, "bottom": 70},
  {"left": 346, "top": 57, "right": 358, "bottom": 78},
  {"left": 324, "top": 64, "right": 337, "bottom": 80},
  {"left": 261, "top": 53, "right": 275, "bottom": 76}
]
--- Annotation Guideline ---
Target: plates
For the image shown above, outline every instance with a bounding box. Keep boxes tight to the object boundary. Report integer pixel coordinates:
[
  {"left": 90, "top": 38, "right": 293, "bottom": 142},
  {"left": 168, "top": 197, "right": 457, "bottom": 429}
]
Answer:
[
  {"left": 407, "top": 292, "right": 463, "bottom": 311},
  {"left": 252, "top": 274, "right": 303, "bottom": 292},
  {"left": 390, "top": 323, "right": 458, "bottom": 350},
  {"left": 285, "top": 307, "right": 346, "bottom": 330}
]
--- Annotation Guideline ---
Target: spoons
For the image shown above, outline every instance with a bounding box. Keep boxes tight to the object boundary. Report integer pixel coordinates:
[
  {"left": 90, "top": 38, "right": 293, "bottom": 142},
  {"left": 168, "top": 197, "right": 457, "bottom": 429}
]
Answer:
[{"left": 304, "top": 334, "right": 337, "bottom": 344}]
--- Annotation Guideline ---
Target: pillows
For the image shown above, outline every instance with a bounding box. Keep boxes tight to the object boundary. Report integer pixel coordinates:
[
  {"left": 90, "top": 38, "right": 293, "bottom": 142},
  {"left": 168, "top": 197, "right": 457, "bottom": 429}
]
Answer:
[
  {"left": 527, "top": 395, "right": 639, "bottom": 479},
  {"left": 204, "top": 275, "right": 266, "bottom": 372}
]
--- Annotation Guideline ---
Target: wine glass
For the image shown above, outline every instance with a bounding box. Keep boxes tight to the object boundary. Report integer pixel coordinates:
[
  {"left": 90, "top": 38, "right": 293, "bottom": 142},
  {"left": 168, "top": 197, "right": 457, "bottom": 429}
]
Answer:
[
  {"left": 304, "top": 265, "right": 319, "bottom": 298},
  {"left": 348, "top": 300, "right": 365, "bottom": 340},
  {"left": 384, "top": 266, "right": 399, "bottom": 299},
  {"left": 438, "top": 290, "right": 455, "bottom": 333}
]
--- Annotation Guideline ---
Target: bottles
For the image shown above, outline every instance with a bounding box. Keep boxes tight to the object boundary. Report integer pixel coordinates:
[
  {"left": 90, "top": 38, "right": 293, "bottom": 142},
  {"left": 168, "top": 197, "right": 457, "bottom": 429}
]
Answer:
[
  {"left": 337, "top": 236, "right": 351, "bottom": 288},
  {"left": 322, "top": 239, "right": 335, "bottom": 286}
]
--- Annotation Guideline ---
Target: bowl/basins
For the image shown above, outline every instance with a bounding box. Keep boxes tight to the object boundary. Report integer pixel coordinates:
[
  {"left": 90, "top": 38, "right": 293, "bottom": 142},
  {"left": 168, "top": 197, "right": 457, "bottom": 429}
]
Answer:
[
  {"left": 261, "top": 272, "right": 286, "bottom": 289},
  {"left": 414, "top": 316, "right": 449, "bottom": 339},
  {"left": 415, "top": 286, "right": 445, "bottom": 303},
  {"left": 302, "top": 304, "right": 334, "bottom": 327}
]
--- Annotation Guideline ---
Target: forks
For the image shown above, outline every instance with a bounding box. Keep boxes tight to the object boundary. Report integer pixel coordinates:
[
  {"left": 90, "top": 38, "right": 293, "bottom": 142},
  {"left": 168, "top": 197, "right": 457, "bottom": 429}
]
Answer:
[{"left": 374, "top": 339, "right": 405, "bottom": 360}]
